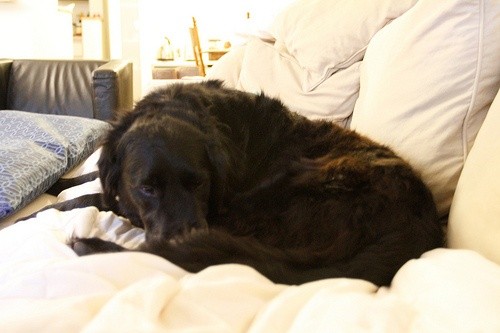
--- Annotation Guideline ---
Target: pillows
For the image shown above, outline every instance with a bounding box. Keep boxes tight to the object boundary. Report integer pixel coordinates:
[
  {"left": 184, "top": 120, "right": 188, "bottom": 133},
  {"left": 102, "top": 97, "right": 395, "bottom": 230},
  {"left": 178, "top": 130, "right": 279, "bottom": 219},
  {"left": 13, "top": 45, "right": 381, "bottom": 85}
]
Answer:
[{"left": 0, "top": 110, "right": 114, "bottom": 220}]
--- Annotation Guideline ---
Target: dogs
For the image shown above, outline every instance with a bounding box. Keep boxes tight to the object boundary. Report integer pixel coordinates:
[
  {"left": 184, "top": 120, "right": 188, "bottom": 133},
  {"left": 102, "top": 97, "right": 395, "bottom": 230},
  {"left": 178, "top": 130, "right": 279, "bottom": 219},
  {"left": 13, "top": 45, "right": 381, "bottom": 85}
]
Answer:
[{"left": 14, "top": 79, "right": 447, "bottom": 287}]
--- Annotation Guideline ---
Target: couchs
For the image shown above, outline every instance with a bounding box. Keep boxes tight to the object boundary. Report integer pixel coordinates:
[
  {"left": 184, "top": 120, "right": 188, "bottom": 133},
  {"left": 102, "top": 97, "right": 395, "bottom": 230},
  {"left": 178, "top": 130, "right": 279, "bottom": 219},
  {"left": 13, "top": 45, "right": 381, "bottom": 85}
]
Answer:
[{"left": 0, "top": 59, "right": 134, "bottom": 122}]
